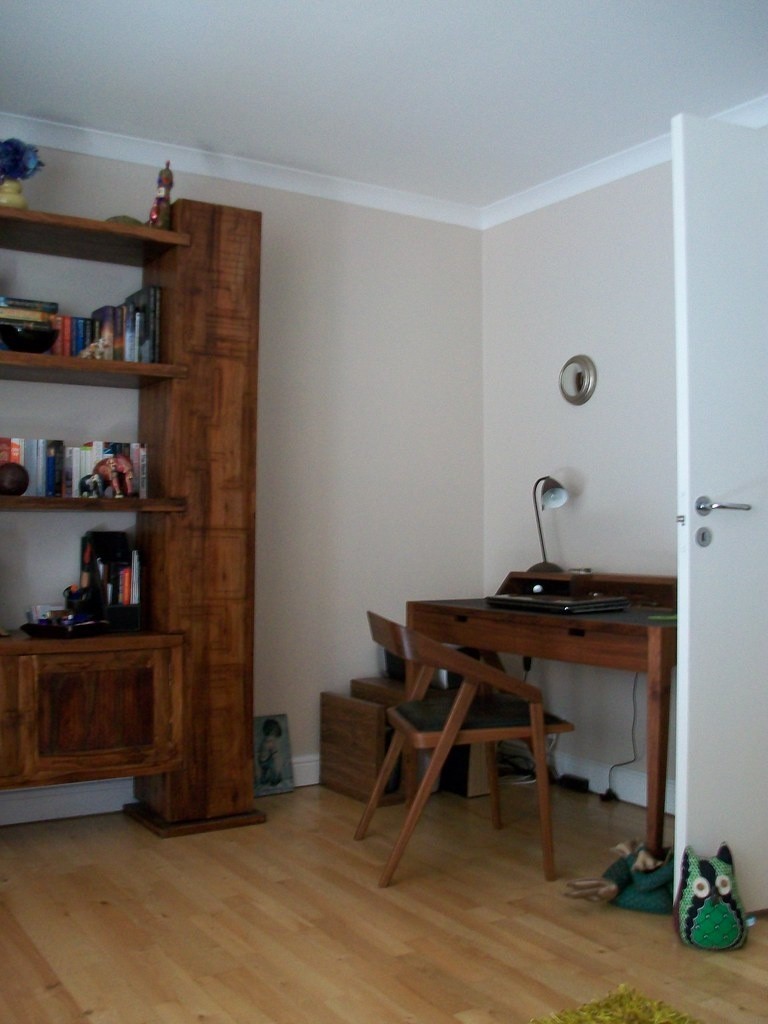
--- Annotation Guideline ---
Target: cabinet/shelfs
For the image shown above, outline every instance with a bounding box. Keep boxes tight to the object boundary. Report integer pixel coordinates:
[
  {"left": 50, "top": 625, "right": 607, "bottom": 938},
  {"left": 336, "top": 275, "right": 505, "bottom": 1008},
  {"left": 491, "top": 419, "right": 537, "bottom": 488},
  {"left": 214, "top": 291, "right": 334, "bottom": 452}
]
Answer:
[
  {"left": 319, "top": 674, "right": 453, "bottom": 807},
  {"left": 0, "top": 197, "right": 265, "bottom": 836}
]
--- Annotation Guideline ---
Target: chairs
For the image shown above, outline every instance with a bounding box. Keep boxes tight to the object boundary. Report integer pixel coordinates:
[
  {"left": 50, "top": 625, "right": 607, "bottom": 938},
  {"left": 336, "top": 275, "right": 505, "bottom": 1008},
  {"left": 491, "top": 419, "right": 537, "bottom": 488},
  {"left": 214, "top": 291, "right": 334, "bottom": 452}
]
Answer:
[{"left": 352, "top": 611, "right": 573, "bottom": 888}]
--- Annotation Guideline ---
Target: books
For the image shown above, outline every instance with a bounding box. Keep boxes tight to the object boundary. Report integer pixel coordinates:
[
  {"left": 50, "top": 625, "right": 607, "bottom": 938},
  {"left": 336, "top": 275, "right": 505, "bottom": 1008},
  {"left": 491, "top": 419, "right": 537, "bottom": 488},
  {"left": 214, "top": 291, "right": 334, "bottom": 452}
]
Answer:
[
  {"left": 95, "top": 550, "right": 139, "bottom": 605},
  {"left": 0, "top": 284, "right": 162, "bottom": 364},
  {"left": 0, "top": 437, "right": 147, "bottom": 499}
]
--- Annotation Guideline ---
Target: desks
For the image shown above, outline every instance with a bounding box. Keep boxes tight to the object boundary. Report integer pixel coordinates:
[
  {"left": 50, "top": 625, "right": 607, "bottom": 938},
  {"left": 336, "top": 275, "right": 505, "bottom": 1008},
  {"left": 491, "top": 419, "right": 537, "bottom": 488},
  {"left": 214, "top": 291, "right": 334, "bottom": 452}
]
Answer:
[{"left": 407, "top": 576, "right": 678, "bottom": 859}]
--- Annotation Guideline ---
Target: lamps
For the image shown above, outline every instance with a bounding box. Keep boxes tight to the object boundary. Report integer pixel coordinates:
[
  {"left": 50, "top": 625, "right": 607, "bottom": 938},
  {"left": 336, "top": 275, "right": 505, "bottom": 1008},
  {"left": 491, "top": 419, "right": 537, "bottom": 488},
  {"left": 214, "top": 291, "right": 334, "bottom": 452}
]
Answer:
[{"left": 525, "top": 477, "right": 570, "bottom": 571}]
